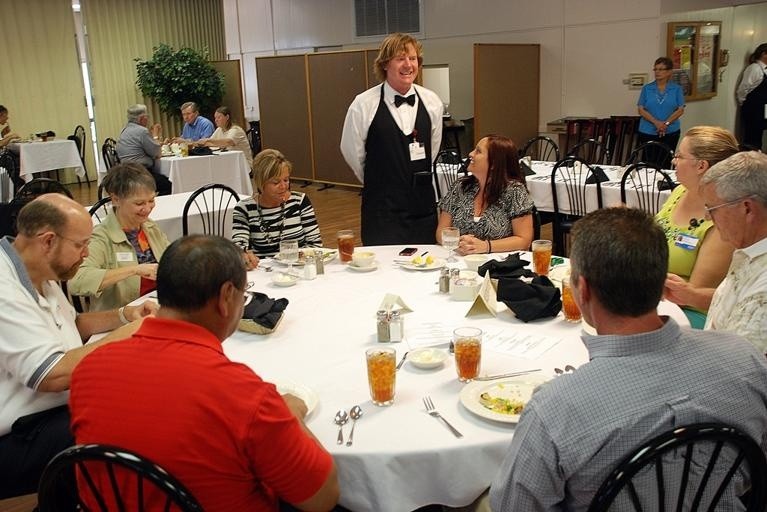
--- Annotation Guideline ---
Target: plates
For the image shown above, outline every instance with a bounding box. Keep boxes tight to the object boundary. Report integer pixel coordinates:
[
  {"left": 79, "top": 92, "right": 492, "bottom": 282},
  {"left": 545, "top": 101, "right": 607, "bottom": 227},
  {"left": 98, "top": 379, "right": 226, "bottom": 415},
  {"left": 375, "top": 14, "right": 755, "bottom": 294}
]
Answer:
[
  {"left": 261, "top": 378, "right": 319, "bottom": 420},
  {"left": 399, "top": 257, "right": 447, "bottom": 271},
  {"left": 459, "top": 373, "right": 552, "bottom": 424},
  {"left": 270, "top": 274, "right": 297, "bottom": 286},
  {"left": 347, "top": 262, "right": 380, "bottom": 272}
]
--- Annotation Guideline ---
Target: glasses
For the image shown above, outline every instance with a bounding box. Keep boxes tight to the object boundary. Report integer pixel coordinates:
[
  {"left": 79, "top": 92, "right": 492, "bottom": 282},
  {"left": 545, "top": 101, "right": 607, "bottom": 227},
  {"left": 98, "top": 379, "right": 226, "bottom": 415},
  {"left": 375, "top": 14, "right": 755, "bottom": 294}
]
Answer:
[
  {"left": 673, "top": 151, "right": 702, "bottom": 163},
  {"left": 704, "top": 194, "right": 759, "bottom": 221},
  {"left": 36, "top": 231, "right": 92, "bottom": 252},
  {"left": 653, "top": 68, "right": 670, "bottom": 72}
]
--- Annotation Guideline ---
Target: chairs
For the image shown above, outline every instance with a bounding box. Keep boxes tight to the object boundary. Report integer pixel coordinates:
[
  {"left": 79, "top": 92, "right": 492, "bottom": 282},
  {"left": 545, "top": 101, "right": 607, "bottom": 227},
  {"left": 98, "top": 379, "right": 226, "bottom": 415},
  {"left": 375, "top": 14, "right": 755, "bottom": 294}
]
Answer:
[
  {"left": 588, "top": 421, "right": 764, "bottom": 511},
  {"left": 420, "top": 131, "right": 681, "bottom": 257},
  {"left": 37, "top": 442, "right": 203, "bottom": 511}
]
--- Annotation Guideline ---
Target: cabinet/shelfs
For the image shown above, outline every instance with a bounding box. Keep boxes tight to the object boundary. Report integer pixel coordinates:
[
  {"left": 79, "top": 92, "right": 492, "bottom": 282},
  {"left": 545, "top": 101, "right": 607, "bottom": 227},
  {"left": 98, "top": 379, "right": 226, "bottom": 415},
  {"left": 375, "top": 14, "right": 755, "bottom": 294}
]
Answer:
[{"left": 665, "top": 20, "right": 723, "bottom": 102}]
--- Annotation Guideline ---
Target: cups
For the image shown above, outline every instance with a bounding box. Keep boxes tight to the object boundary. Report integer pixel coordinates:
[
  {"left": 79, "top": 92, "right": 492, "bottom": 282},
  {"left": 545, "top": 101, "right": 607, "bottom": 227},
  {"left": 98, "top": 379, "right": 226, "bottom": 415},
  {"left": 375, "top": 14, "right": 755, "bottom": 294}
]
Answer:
[
  {"left": 561, "top": 278, "right": 582, "bottom": 323},
  {"left": 352, "top": 252, "right": 375, "bottom": 267},
  {"left": 365, "top": 346, "right": 396, "bottom": 406},
  {"left": 531, "top": 239, "right": 552, "bottom": 277},
  {"left": 337, "top": 229, "right": 353, "bottom": 264},
  {"left": 181, "top": 143, "right": 188, "bottom": 157},
  {"left": 452, "top": 325, "right": 482, "bottom": 383}
]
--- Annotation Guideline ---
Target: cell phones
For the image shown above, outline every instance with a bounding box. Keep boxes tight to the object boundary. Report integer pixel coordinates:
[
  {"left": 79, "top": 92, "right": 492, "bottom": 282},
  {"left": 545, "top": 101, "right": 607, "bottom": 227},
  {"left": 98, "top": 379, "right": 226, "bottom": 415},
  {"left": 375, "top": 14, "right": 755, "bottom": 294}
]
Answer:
[{"left": 400, "top": 248, "right": 417, "bottom": 255}]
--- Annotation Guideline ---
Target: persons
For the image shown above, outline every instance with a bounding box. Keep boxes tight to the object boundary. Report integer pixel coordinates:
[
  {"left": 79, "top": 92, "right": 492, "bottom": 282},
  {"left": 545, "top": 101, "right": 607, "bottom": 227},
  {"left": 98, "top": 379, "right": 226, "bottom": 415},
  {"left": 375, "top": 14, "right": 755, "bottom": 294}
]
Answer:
[
  {"left": 636, "top": 56, "right": 687, "bottom": 169},
  {"left": 112, "top": 103, "right": 173, "bottom": 195},
  {"left": 435, "top": 133, "right": 536, "bottom": 256},
  {"left": 439, "top": 205, "right": 766, "bottom": 512},
  {"left": 661, "top": 149, "right": 766, "bottom": 360},
  {"left": 683, "top": 45, "right": 711, "bottom": 73},
  {"left": 230, "top": 147, "right": 323, "bottom": 273},
  {"left": 196, "top": 105, "right": 255, "bottom": 180},
  {"left": 0, "top": 192, "right": 168, "bottom": 511},
  {"left": 65, "top": 159, "right": 174, "bottom": 312},
  {"left": 66, "top": 233, "right": 341, "bottom": 512},
  {"left": 736, "top": 42, "right": 767, "bottom": 152},
  {"left": 171, "top": 101, "right": 215, "bottom": 146},
  {"left": 651, "top": 125, "right": 742, "bottom": 332},
  {"left": 339, "top": 30, "right": 445, "bottom": 245},
  {"left": 0, "top": 104, "right": 32, "bottom": 196}
]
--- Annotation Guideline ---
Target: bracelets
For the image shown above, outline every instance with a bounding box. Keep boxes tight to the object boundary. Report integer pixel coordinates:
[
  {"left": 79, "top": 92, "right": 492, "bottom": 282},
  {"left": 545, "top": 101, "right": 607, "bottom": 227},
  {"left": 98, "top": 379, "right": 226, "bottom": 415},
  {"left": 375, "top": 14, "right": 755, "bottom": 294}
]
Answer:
[
  {"left": 153, "top": 136, "right": 158, "bottom": 141},
  {"left": 663, "top": 120, "right": 671, "bottom": 126},
  {"left": 117, "top": 305, "right": 133, "bottom": 325},
  {"left": 484, "top": 239, "right": 491, "bottom": 255}
]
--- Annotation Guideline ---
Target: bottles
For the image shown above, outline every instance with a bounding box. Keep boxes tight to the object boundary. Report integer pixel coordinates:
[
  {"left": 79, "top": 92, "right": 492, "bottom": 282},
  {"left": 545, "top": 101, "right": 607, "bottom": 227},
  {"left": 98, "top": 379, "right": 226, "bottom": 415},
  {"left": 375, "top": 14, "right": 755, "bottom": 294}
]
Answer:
[
  {"left": 389, "top": 309, "right": 402, "bottom": 343},
  {"left": 302, "top": 250, "right": 323, "bottom": 280},
  {"left": 439, "top": 269, "right": 448, "bottom": 294},
  {"left": 377, "top": 311, "right": 388, "bottom": 343},
  {"left": 449, "top": 268, "right": 459, "bottom": 295}
]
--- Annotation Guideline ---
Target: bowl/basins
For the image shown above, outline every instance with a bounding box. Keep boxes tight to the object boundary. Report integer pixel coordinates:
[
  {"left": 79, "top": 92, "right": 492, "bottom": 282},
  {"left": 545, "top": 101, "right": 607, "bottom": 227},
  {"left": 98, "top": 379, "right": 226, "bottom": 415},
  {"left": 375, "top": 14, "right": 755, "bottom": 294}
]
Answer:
[
  {"left": 462, "top": 255, "right": 488, "bottom": 271},
  {"left": 406, "top": 347, "right": 448, "bottom": 370}
]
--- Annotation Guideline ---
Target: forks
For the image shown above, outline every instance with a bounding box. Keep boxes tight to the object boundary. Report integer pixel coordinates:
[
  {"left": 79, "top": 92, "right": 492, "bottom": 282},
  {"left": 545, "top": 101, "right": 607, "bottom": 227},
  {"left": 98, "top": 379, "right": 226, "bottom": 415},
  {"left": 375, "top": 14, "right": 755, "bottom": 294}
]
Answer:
[{"left": 420, "top": 395, "right": 463, "bottom": 438}]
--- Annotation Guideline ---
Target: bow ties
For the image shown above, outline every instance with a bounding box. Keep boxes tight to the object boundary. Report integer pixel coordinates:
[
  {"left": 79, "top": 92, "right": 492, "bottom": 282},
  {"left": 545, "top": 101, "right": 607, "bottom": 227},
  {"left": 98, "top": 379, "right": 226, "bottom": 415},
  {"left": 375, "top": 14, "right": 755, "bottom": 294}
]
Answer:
[{"left": 395, "top": 94, "right": 415, "bottom": 108}]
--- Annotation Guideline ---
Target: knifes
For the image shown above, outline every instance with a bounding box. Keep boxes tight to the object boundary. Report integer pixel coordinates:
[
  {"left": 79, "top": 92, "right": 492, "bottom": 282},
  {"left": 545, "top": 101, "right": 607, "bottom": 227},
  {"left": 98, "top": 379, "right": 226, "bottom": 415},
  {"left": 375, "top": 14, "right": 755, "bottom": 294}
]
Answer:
[
  {"left": 397, "top": 352, "right": 409, "bottom": 370},
  {"left": 473, "top": 368, "right": 541, "bottom": 382}
]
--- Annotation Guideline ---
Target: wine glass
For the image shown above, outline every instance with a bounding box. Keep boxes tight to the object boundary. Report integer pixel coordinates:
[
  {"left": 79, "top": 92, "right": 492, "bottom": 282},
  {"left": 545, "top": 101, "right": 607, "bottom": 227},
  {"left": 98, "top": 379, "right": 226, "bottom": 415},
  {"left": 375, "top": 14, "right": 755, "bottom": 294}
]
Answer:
[
  {"left": 279, "top": 240, "right": 300, "bottom": 280},
  {"left": 441, "top": 227, "right": 460, "bottom": 264}
]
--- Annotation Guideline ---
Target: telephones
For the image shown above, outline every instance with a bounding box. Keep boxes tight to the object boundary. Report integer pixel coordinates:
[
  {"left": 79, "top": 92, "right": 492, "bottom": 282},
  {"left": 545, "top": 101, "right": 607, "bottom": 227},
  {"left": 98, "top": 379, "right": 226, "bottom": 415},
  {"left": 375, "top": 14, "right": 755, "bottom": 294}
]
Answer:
[{"left": 720, "top": 48, "right": 729, "bottom": 67}]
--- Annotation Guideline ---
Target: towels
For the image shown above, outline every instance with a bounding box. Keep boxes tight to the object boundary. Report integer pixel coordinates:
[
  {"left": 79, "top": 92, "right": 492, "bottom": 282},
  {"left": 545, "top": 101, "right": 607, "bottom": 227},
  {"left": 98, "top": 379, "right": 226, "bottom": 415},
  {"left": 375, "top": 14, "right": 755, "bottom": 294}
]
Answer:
[
  {"left": 495, "top": 274, "right": 563, "bottom": 324},
  {"left": 477, "top": 252, "right": 531, "bottom": 281},
  {"left": 241, "top": 291, "right": 290, "bottom": 328}
]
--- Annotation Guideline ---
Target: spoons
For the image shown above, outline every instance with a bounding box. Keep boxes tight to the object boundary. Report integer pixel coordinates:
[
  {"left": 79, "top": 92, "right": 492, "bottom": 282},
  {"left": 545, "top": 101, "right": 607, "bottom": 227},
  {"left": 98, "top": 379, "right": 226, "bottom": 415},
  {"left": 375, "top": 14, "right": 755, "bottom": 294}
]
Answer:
[
  {"left": 346, "top": 405, "right": 362, "bottom": 446},
  {"left": 334, "top": 410, "right": 348, "bottom": 445}
]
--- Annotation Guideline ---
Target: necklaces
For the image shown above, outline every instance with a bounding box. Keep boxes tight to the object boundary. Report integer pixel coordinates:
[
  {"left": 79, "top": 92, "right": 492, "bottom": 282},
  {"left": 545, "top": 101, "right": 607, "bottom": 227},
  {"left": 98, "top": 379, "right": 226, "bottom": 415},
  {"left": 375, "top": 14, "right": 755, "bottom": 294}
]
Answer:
[{"left": 255, "top": 192, "right": 286, "bottom": 246}]
